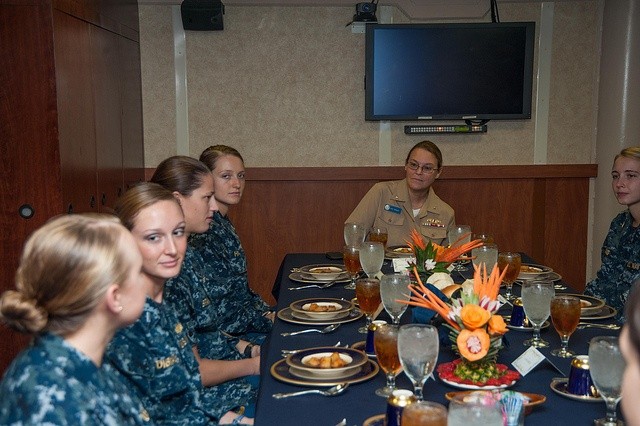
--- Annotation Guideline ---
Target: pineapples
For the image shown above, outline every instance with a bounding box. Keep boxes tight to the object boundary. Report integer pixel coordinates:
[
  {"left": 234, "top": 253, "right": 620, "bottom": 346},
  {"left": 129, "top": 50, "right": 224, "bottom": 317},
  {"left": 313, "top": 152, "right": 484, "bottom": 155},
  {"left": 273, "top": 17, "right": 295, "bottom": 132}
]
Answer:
[{"left": 442, "top": 315, "right": 507, "bottom": 384}]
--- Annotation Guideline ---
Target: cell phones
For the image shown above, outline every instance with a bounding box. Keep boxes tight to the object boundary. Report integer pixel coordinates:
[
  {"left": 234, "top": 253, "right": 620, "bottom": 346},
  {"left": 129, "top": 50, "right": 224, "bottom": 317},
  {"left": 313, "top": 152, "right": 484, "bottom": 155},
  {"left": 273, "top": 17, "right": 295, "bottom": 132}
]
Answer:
[{"left": 327, "top": 252, "right": 343, "bottom": 260}]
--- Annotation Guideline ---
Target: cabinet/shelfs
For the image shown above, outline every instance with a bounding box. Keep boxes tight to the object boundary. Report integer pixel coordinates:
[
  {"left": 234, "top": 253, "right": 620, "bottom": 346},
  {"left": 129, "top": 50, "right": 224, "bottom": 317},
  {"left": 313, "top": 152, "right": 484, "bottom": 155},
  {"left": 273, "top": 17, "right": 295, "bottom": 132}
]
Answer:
[{"left": 1, "top": 0, "right": 145, "bottom": 372}]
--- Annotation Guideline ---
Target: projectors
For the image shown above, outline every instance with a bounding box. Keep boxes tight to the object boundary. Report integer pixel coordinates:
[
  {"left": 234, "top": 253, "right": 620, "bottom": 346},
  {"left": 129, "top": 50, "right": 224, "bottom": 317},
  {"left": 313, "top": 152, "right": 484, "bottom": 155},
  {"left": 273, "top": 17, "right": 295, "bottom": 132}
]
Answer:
[{"left": 354, "top": 2, "right": 378, "bottom": 20}]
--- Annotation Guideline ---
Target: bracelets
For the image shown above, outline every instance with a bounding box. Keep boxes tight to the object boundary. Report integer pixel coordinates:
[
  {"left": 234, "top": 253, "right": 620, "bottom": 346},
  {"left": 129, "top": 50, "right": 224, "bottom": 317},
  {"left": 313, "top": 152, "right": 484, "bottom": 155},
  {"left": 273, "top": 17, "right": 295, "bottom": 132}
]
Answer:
[
  {"left": 233, "top": 414, "right": 243, "bottom": 425},
  {"left": 270, "top": 312, "right": 275, "bottom": 321}
]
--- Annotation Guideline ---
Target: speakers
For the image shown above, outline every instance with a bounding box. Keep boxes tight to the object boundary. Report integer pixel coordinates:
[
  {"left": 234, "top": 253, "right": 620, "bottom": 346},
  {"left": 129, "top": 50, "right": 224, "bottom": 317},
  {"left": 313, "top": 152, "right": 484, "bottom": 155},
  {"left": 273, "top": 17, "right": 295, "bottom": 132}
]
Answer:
[{"left": 180, "top": 0, "right": 225, "bottom": 31}]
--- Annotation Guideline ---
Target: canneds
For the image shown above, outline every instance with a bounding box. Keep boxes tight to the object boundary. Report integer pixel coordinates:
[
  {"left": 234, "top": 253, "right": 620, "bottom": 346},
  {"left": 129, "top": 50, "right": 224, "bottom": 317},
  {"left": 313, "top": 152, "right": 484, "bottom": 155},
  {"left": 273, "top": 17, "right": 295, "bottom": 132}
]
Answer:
[
  {"left": 387, "top": 390, "right": 416, "bottom": 425},
  {"left": 510, "top": 298, "right": 527, "bottom": 327},
  {"left": 569, "top": 354, "right": 594, "bottom": 396},
  {"left": 367, "top": 321, "right": 388, "bottom": 353}
]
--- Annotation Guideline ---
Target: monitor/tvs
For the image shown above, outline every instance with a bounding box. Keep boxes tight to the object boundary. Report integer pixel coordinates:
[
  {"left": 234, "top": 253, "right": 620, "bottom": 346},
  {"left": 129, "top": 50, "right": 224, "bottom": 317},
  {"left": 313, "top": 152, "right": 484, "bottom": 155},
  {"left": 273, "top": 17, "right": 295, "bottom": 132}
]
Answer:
[{"left": 364, "top": 22, "right": 535, "bottom": 122}]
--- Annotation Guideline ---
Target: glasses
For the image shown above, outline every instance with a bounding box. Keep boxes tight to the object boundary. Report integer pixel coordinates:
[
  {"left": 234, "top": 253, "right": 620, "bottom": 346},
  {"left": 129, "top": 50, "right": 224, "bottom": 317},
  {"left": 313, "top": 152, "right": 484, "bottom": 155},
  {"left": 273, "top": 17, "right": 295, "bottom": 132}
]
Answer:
[{"left": 406, "top": 159, "right": 439, "bottom": 173}]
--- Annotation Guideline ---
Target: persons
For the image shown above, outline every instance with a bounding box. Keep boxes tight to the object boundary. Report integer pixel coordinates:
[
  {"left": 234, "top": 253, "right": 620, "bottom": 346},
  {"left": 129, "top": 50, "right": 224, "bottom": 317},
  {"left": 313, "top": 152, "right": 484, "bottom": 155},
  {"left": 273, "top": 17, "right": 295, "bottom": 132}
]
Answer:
[
  {"left": 619, "top": 279, "right": 640, "bottom": 425},
  {"left": 0, "top": 213, "right": 157, "bottom": 426},
  {"left": 148, "top": 154, "right": 266, "bottom": 387},
  {"left": 344, "top": 140, "right": 457, "bottom": 248},
  {"left": 582, "top": 146, "right": 639, "bottom": 318},
  {"left": 199, "top": 144, "right": 277, "bottom": 323},
  {"left": 106, "top": 178, "right": 254, "bottom": 425}
]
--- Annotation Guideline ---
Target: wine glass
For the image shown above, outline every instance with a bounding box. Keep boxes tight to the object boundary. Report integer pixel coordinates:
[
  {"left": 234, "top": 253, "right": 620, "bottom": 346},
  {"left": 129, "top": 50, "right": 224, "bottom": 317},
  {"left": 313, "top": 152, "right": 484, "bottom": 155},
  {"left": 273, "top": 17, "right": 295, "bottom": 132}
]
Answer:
[
  {"left": 448, "top": 224, "right": 471, "bottom": 246},
  {"left": 402, "top": 402, "right": 446, "bottom": 426},
  {"left": 550, "top": 296, "right": 581, "bottom": 356},
  {"left": 476, "top": 235, "right": 493, "bottom": 243},
  {"left": 345, "top": 245, "right": 359, "bottom": 289},
  {"left": 381, "top": 276, "right": 410, "bottom": 323},
  {"left": 377, "top": 324, "right": 401, "bottom": 396},
  {"left": 360, "top": 241, "right": 384, "bottom": 278},
  {"left": 497, "top": 253, "right": 522, "bottom": 299},
  {"left": 345, "top": 222, "right": 365, "bottom": 246},
  {"left": 522, "top": 279, "right": 556, "bottom": 347},
  {"left": 400, "top": 323, "right": 439, "bottom": 401},
  {"left": 447, "top": 393, "right": 504, "bottom": 426},
  {"left": 369, "top": 227, "right": 388, "bottom": 245},
  {"left": 588, "top": 337, "right": 626, "bottom": 426},
  {"left": 356, "top": 278, "right": 381, "bottom": 332},
  {"left": 472, "top": 247, "right": 498, "bottom": 280}
]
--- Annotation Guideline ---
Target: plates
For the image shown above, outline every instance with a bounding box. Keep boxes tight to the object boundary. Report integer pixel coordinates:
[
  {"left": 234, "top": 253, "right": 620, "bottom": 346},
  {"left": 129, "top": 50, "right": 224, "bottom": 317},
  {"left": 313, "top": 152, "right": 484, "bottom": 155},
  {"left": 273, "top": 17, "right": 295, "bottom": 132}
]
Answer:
[
  {"left": 556, "top": 291, "right": 604, "bottom": 311},
  {"left": 271, "top": 357, "right": 379, "bottom": 386},
  {"left": 289, "top": 299, "right": 353, "bottom": 318},
  {"left": 517, "top": 264, "right": 554, "bottom": 279},
  {"left": 362, "top": 414, "right": 385, "bottom": 425},
  {"left": 300, "top": 265, "right": 348, "bottom": 277},
  {"left": 277, "top": 307, "right": 363, "bottom": 324},
  {"left": 445, "top": 391, "right": 546, "bottom": 416},
  {"left": 285, "top": 346, "right": 367, "bottom": 375},
  {"left": 354, "top": 341, "right": 377, "bottom": 357},
  {"left": 388, "top": 244, "right": 416, "bottom": 254},
  {"left": 504, "top": 315, "right": 549, "bottom": 333},
  {"left": 515, "top": 272, "right": 562, "bottom": 285},
  {"left": 579, "top": 306, "right": 619, "bottom": 318},
  {"left": 551, "top": 378, "right": 604, "bottom": 403},
  {"left": 384, "top": 253, "right": 415, "bottom": 259},
  {"left": 288, "top": 272, "right": 360, "bottom": 283},
  {"left": 439, "top": 370, "right": 517, "bottom": 389}
]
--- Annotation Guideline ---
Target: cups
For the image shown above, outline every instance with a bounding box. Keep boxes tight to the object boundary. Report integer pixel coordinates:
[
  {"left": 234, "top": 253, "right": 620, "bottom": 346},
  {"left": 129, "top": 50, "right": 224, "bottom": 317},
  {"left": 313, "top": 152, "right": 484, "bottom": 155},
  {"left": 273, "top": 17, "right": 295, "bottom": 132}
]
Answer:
[
  {"left": 365, "top": 320, "right": 385, "bottom": 353},
  {"left": 510, "top": 300, "right": 529, "bottom": 327},
  {"left": 569, "top": 356, "right": 590, "bottom": 394}
]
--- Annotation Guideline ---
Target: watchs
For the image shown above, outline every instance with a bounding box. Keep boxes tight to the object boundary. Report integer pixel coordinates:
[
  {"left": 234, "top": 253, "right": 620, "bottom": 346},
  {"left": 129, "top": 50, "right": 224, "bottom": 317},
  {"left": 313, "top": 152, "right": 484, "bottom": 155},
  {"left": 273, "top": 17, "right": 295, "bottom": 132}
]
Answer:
[{"left": 245, "top": 343, "right": 254, "bottom": 360}]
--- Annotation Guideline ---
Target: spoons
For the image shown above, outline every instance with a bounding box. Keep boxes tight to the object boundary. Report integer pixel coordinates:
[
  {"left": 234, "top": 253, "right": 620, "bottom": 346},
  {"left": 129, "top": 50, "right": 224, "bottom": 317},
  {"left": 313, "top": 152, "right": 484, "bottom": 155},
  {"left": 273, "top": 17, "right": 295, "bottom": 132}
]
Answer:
[
  {"left": 272, "top": 383, "right": 347, "bottom": 399},
  {"left": 288, "top": 281, "right": 335, "bottom": 290},
  {"left": 283, "top": 324, "right": 340, "bottom": 337}
]
